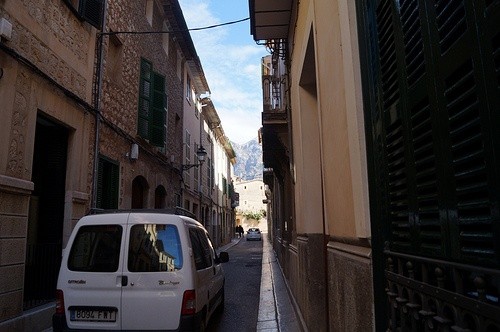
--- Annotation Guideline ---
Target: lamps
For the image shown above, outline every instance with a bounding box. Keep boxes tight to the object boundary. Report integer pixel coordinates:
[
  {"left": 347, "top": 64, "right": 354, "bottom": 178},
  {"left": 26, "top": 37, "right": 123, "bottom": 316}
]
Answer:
[{"left": 171, "top": 138, "right": 208, "bottom": 179}]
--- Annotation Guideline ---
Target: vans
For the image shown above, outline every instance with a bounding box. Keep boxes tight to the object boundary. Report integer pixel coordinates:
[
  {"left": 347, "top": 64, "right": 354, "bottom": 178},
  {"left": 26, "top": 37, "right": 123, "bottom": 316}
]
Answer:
[{"left": 50, "top": 204, "right": 231, "bottom": 332}]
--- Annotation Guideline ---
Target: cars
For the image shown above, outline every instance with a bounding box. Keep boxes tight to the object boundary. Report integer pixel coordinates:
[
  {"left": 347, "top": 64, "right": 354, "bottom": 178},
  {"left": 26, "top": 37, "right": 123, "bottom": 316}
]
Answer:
[{"left": 246, "top": 227, "right": 262, "bottom": 241}]
